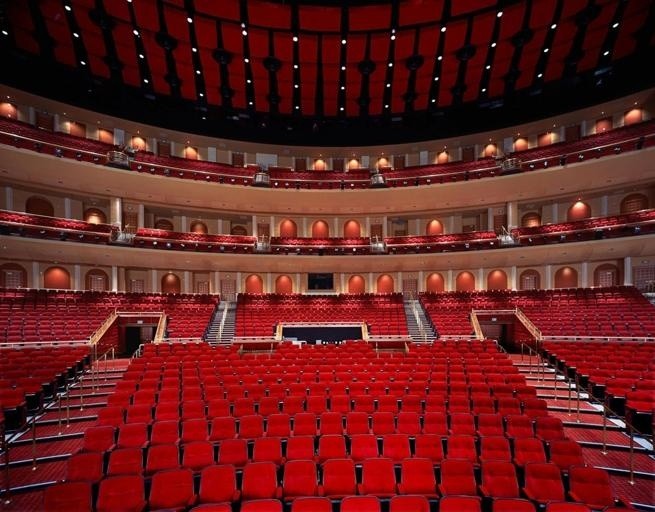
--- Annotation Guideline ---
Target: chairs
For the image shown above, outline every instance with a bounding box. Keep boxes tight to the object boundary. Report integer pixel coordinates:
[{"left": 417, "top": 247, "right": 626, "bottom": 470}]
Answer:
[
  {"left": 39, "top": 341, "right": 637, "bottom": 511},
  {"left": 130, "top": 148, "right": 261, "bottom": 186},
  {"left": 2, "top": 114, "right": 123, "bottom": 170},
  {"left": 379, "top": 153, "right": 501, "bottom": 188},
  {"left": 271, "top": 235, "right": 372, "bottom": 248},
  {"left": 417, "top": 283, "right": 654, "bottom": 337},
  {"left": 1, "top": 346, "right": 93, "bottom": 442},
  {"left": 3, "top": 209, "right": 121, "bottom": 244},
  {"left": 511, "top": 116, "right": 655, "bottom": 171},
  {"left": 268, "top": 166, "right": 371, "bottom": 186},
  {"left": 232, "top": 291, "right": 411, "bottom": 341},
  {"left": 383, "top": 231, "right": 499, "bottom": 245},
  {"left": 529, "top": 340, "right": 655, "bottom": 454},
  {"left": 0, "top": 285, "right": 222, "bottom": 344},
  {"left": 510, "top": 207, "right": 655, "bottom": 241},
  {"left": 136, "top": 226, "right": 259, "bottom": 252}
]
